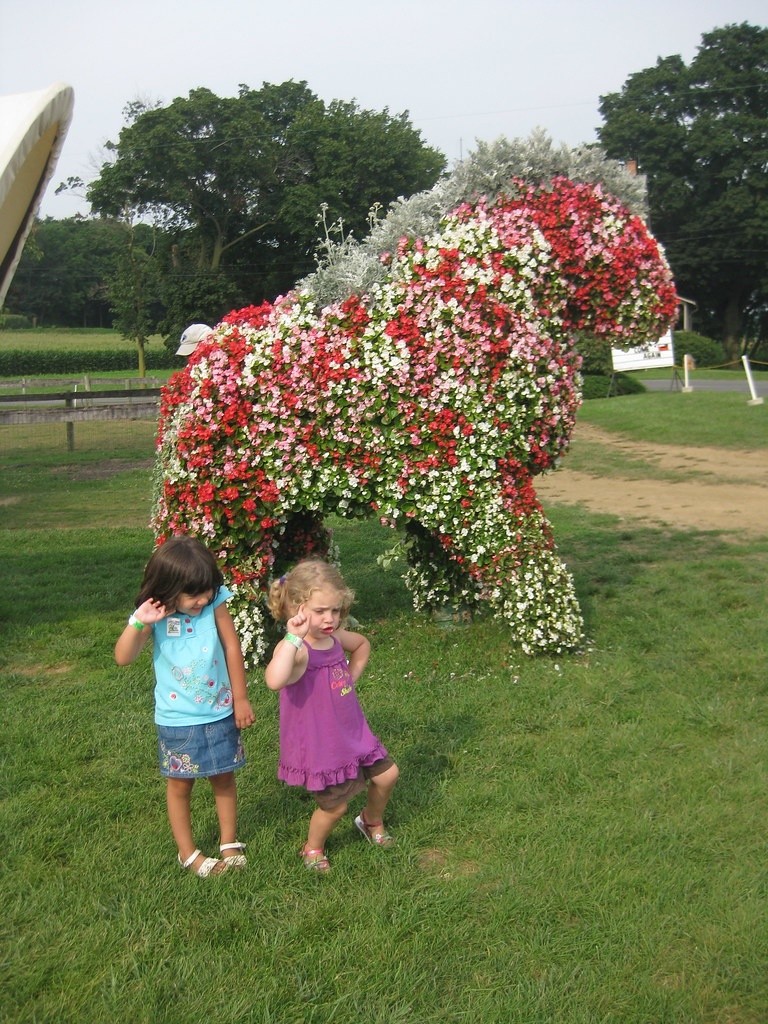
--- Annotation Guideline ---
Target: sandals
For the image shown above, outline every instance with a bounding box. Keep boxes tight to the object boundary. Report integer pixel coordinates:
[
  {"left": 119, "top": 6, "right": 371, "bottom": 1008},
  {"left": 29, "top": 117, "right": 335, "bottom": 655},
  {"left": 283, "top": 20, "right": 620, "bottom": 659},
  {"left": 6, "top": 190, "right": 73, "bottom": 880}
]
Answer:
[
  {"left": 354, "top": 808, "right": 394, "bottom": 848},
  {"left": 220, "top": 838, "right": 248, "bottom": 868},
  {"left": 300, "top": 838, "right": 330, "bottom": 872},
  {"left": 177, "top": 849, "right": 229, "bottom": 879}
]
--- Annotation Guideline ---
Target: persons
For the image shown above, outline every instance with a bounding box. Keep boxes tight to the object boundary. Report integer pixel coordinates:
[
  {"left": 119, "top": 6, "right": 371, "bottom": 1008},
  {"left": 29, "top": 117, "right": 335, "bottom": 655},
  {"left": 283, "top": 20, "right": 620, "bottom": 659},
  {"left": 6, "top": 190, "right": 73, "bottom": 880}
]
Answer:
[
  {"left": 176, "top": 324, "right": 212, "bottom": 362},
  {"left": 264, "top": 562, "right": 399, "bottom": 873},
  {"left": 115, "top": 537, "right": 256, "bottom": 877}
]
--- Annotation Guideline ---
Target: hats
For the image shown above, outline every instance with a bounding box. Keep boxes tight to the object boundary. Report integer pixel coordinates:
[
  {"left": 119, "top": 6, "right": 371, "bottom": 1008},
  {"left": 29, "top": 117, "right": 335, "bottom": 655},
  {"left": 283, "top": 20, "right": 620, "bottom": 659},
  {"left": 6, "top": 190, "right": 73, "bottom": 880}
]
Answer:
[{"left": 175, "top": 324, "right": 212, "bottom": 356}]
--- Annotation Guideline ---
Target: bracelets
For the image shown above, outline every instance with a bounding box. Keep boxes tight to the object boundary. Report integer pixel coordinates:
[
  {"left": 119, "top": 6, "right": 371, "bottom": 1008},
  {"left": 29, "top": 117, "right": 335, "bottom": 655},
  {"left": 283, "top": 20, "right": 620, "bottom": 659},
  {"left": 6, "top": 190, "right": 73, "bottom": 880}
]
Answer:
[
  {"left": 285, "top": 633, "right": 304, "bottom": 648},
  {"left": 129, "top": 616, "right": 146, "bottom": 631}
]
